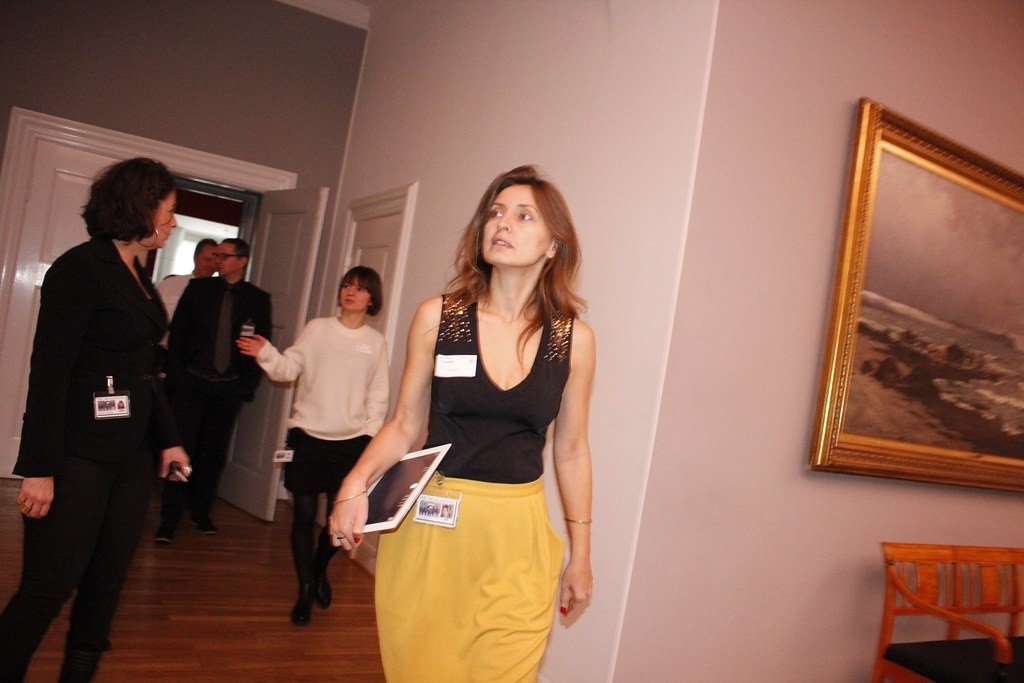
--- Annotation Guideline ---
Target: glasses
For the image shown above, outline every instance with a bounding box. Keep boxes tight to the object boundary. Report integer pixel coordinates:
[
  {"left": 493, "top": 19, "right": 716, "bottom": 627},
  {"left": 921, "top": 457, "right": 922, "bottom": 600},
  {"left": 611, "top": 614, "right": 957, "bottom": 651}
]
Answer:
[{"left": 212, "top": 253, "right": 243, "bottom": 260}]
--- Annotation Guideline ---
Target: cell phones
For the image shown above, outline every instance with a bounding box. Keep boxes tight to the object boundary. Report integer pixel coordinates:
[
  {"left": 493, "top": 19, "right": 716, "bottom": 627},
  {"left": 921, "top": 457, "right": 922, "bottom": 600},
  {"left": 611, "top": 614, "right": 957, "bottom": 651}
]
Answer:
[{"left": 172, "top": 462, "right": 188, "bottom": 483}]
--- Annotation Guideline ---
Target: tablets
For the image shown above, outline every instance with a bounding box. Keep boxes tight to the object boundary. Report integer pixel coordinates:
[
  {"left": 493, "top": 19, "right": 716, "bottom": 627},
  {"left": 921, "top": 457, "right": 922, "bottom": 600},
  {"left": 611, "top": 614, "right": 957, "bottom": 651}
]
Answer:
[{"left": 364, "top": 442, "right": 452, "bottom": 534}]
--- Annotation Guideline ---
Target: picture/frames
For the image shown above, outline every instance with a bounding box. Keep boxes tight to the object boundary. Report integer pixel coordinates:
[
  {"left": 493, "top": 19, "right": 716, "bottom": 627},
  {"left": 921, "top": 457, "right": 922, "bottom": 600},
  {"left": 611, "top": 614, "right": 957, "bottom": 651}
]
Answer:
[{"left": 806, "top": 96, "right": 1024, "bottom": 494}]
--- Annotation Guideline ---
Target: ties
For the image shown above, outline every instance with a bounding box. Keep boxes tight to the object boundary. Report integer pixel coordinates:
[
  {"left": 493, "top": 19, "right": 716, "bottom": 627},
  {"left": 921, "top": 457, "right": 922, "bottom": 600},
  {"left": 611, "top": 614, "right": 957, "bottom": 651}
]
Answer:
[{"left": 214, "top": 285, "right": 232, "bottom": 375}]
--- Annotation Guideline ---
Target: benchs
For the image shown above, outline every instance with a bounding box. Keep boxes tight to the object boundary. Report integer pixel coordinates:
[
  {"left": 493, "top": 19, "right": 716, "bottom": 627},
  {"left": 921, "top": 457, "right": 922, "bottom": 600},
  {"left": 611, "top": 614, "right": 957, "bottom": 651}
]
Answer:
[{"left": 870, "top": 541, "right": 1024, "bottom": 683}]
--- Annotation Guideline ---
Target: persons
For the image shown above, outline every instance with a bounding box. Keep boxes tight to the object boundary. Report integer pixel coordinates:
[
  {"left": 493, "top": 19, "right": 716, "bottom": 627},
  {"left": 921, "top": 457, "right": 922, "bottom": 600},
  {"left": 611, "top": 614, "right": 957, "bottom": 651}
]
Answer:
[
  {"left": 327, "top": 165, "right": 593, "bottom": 683},
  {"left": 236, "top": 265, "right": 390, "bottom": 628},
  {"left": 0, "top": 155, "right": 193, "bottom": 683},
  {"left": 156, "top": 238, "right": 272, "bottom": 546},
  {"left": 156, "top": 240, "right": 221, "bottom": 347}
]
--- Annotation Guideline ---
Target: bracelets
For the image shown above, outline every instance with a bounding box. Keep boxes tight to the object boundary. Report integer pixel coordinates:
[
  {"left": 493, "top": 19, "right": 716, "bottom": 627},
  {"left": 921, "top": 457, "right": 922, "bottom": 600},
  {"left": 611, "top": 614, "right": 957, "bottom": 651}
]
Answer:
[
  {"left": 564, "top": 518, "right": 593, "bottom": 525},
  {"left": 333, "top": 490, "right": 368, "bottom": 503}
]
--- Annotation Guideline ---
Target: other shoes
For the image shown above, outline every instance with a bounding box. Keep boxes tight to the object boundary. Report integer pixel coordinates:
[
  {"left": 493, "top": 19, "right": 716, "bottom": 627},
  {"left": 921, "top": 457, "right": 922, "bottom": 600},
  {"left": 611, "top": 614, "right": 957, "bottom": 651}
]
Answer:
[
  {"left": 292, "top": 582, "right": 313, "bottom": 625},
  {"left": 190, "top": 514, "right": 217, "bottom": 534},
  {"left": 154, "top": 519, "right": 176, "bottom": 543},
  {"left": 315, "top": 572, "right": 331, "bottom": 608}
]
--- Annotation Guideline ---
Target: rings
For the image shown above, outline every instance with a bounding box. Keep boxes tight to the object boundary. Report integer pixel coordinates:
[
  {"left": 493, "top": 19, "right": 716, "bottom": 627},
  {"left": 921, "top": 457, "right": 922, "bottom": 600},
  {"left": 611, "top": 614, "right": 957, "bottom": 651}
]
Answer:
[
  {"left": 19, "top": 504, "right": 30, "bottom": 514},
  {"left": 337, "top": 536, "right": 345, "bottom": 539}
]
